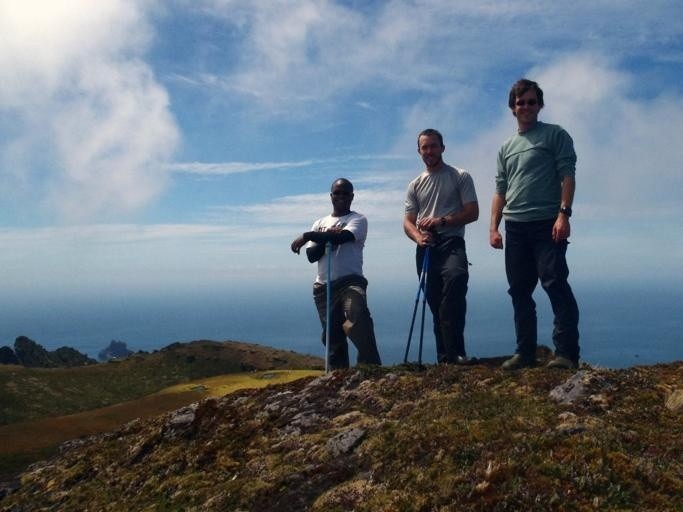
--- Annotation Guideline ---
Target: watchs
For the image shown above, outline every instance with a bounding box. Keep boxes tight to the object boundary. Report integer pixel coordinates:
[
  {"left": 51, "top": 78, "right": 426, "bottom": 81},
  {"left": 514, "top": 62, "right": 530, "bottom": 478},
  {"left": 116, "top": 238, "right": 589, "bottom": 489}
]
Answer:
[
  {"left": 438, "top": 215, "right": 447, "bottom": 227},
  {"left": 559, "top": 205, "right": 572, "bottom": 216}
]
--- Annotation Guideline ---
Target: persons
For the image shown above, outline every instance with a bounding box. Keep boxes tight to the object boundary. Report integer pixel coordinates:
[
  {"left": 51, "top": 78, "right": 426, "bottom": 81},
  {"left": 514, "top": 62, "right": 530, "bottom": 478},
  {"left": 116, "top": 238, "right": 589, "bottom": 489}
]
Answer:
[
  {"left": 403, "top": 128, "right": 478, "bottom": 365},
  {"left": 291, "top": 178, "right": 381, "bottom": 373},
  {"left": 489, "top": 79, "right": 579, "bottom": 369}
]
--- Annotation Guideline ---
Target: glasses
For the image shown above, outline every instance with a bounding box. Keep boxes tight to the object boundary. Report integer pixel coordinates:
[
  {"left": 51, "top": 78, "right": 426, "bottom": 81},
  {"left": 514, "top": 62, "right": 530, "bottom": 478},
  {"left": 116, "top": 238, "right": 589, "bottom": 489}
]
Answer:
[
  {"left": 333, "top": 188, "right": 352, "bottom": 196},
  {"left": 516, "top": 98, "right": 538, "bottom": 106}
]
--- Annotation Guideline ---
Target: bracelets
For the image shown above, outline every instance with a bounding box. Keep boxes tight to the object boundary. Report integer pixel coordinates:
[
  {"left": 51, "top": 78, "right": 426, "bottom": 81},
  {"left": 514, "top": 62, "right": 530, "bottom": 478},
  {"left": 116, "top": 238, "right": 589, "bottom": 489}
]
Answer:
[{"left": 302, "top": 230, "right": 312, "bottom": 241}]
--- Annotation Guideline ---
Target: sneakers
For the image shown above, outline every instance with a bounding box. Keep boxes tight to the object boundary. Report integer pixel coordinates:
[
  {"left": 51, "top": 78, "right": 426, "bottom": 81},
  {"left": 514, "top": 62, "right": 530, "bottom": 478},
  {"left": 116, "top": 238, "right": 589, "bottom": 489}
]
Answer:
[
  {"left": 500, "top": 352, "right": 538, "bottom": 372},
  {"left": 450, "top": 352, "right": 479, "bottom": 366},
  {"left": 547, "top": 355, "right": 580, "bottom": 371}
]
